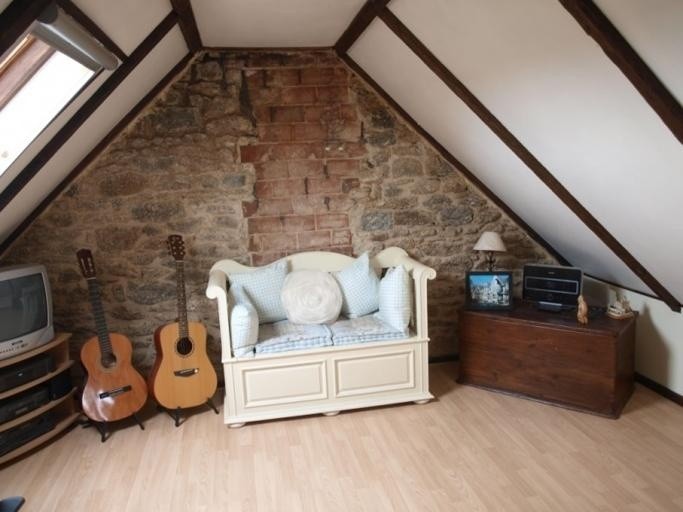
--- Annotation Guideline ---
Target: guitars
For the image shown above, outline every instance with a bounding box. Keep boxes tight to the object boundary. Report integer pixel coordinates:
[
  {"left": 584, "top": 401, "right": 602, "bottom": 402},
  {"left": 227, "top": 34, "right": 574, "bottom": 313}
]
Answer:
[
  {"left": 148, "top": 235, "right": 218, "bottom": 411},
  {"left": 77, "top": 248, "right": 148, "bottom": 423}
]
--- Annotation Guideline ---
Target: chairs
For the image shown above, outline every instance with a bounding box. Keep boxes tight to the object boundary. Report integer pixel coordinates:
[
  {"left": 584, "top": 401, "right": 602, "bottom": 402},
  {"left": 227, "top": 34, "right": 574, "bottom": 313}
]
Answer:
[{"left": 0, "top": 496, "right": 25, "bottom": 512}]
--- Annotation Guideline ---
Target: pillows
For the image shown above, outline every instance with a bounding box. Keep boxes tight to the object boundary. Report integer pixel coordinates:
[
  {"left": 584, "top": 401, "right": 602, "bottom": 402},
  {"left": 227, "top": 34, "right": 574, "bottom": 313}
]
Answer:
[
  {"left": 227, "top": 283, "right": 259, "bottom": 357},
  {"left": 281, "top": 268, "right": 342, "bottom": 325},
  {"left": 331, "top": 251, "right": 381, "bottom": 319},
  {"left": 226, "top": 260, "right": 290, "bottom": 324},
  {"left": 379, "top": 266, "right": 413, "bottom": 331}
]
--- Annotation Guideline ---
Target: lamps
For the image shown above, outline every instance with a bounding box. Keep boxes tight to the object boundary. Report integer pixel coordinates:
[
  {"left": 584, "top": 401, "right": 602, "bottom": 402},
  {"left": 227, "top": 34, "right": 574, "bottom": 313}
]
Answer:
[{"left": 472, "top": 231, "right": 507, "bottom": 272}]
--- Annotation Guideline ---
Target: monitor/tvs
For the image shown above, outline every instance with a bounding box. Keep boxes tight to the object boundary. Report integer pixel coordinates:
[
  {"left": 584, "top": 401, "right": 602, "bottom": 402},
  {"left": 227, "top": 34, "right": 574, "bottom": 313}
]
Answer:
[{"left": 0, "top": 263, "right": 55, "bottom": 361}]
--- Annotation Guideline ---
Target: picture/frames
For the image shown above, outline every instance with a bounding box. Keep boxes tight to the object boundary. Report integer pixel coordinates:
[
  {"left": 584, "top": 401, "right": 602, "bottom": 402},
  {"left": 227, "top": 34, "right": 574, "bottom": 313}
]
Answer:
[{"left": 463, "top": 270, "right": 513, "bottom": 312}]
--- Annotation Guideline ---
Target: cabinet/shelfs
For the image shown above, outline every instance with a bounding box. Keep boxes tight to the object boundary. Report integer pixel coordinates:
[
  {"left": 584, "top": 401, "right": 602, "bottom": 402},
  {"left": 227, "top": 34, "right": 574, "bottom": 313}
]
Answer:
[
  {"left": 0, "top": 331, "right": 81, "bottom": 465},
  {"left": 455, "top": 298, "right": 639, "bottom": 421}
]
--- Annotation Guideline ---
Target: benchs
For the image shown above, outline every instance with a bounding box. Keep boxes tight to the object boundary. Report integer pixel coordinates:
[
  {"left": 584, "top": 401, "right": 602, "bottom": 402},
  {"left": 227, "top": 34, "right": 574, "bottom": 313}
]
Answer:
[{"left": 205, "top": 246, "right": 437, "bottom": 428}]
